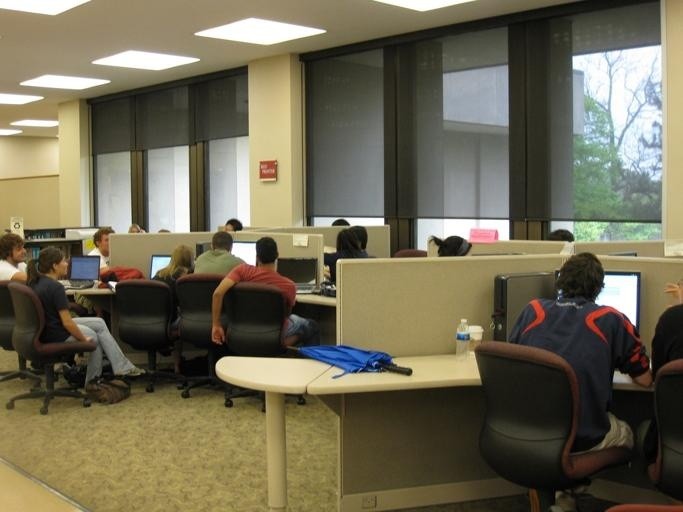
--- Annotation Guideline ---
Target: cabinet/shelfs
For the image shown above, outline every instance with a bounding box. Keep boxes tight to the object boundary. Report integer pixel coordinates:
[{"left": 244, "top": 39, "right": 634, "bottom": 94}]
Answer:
[{"left": 5, "top": 226, "right": 112, "bottom": 280}]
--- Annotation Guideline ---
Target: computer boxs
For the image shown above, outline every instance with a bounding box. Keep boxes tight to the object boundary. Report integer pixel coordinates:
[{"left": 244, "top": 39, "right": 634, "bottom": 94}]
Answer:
[
  {"left": 494, "top": 273, "right": 555, "bottom": 343},
  {"left": 195, "top": 242, "right": 212, "bottom": 258}
]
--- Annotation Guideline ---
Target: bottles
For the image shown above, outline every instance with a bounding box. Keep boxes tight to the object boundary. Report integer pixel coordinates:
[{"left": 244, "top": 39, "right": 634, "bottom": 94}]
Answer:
[{"left": 454, "top": 318, "right": 472, "bottom": 366}]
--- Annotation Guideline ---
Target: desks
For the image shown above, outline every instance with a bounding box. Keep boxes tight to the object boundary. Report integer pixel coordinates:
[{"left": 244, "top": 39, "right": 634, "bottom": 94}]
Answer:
[
  {"left": 306, "top": 348, "right": 656, "bottom": 512},
  {"left": 216, "top": 354, "right": 336, "bottom": 510}
]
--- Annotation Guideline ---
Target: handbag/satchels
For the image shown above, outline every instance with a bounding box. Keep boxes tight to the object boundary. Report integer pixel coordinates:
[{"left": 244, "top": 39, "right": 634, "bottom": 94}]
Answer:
[{"left": 90, "top": 379, "right": 132, "bottom": 404}]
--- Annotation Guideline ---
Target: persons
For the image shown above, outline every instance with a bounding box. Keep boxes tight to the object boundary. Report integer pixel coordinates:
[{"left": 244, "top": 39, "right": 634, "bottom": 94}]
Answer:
[
  {"left": 224, "top": 218, "right": 242, "bottom": 232},
  {"left": 509, "top": 251, "right": 652, "bottom": 511},
  {"left": 210, "top": 235, "right": 321, "bottom": 356},
  {"left": 350, "top": 225, "right": 367, "bottom": 259},
  {"left": 636, "top": 279, "right": 683, "bottom": 486},
  {"left": 71, "top": 225, "right": 116, "bottom": 321},
  {"left": 329, "top": 230, "right": 368, "bottom": 284},
  {"left": 0, "top": 233, "right": 29, "bottom": 283},
  {"left": 23, "top": 246, "right": 152, "bottom": 395},
  {"left": 193, "top": 232, "right": 246, "bottom": 276},
  {"left": 152, "top": 243, "right": 195, "bottom": 375}
]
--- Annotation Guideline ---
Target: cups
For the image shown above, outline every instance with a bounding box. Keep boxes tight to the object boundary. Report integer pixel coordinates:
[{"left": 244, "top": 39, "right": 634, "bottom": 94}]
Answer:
[{"left": 467, "top": 324, "right": 485, "bottom": 359}]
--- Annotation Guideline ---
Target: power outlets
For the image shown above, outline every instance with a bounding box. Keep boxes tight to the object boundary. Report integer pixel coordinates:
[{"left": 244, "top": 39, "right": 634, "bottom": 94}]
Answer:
[{"left": 362, "top": 495, "right": 376, "bottom": 508}]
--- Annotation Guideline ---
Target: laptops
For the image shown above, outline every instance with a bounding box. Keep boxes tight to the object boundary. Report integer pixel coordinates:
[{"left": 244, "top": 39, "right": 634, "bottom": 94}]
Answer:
[
  {"left": 227, "top": 240, "right": 258, "bottom": 267},
  {"left": 277, "top": 258, "right": 319, "bottom": 294},
  {"left": 149, "top": 254, "right": 178, "bottom": 281}
]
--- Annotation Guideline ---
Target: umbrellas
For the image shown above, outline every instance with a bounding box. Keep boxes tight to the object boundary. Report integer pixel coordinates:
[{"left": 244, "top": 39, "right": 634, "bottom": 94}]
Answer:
[{"left": 286, "top": 342, "right": 414, "bottom": 379}]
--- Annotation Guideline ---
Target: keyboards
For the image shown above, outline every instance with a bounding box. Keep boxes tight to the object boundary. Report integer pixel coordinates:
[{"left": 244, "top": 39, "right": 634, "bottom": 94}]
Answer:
[{"left": 60, "top": 280, "right": 94, "bottom": 288}]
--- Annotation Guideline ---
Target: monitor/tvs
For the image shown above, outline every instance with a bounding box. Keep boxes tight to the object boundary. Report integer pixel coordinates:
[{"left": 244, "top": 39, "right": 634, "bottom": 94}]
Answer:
[
  {"left": 69, "top": 255, "right": 101, "bottom": 279},
  {"left": 552, "top": 269, "right": 640, "bottom": 336}
]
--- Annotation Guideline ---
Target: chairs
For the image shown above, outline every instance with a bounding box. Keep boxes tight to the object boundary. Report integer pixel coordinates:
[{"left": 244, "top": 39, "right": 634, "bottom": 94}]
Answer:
[
  {"left": 476, "top": 340, "right": 634, "bottom": 512},
  {"left": 0, "top": 274, "right": 321, "bottom": 414},
  {"left": 647, "top": 358, "right": 683, "bottom": 503}
]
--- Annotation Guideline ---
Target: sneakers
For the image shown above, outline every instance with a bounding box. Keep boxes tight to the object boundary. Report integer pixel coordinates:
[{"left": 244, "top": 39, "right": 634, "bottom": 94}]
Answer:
[
  {"left": 84, "top": 382, "right": 101, "bottom": 392},
  {"left": 113, "top": 365, "right": 146, "bottom": 378}
]
